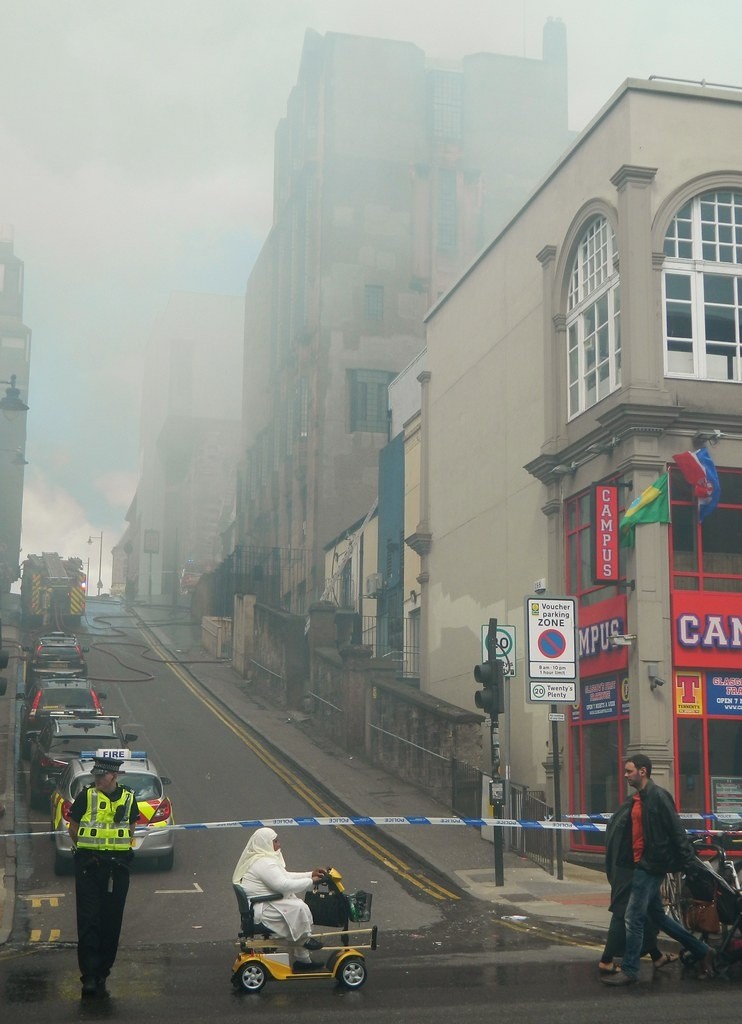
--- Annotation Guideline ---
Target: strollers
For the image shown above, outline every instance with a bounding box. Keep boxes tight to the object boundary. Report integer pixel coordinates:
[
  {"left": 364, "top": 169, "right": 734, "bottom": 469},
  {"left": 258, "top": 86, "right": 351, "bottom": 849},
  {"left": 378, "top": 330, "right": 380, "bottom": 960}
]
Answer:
[{"left": 668, "top": 837, "right": 742, "bottom": 974}]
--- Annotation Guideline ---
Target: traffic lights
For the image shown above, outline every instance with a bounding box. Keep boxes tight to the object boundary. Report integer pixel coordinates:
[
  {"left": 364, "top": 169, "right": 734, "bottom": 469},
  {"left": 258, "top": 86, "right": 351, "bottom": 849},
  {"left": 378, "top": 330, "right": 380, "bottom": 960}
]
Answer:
[{"left": 473, "top": 658, "right": 505, "bottom": 715}]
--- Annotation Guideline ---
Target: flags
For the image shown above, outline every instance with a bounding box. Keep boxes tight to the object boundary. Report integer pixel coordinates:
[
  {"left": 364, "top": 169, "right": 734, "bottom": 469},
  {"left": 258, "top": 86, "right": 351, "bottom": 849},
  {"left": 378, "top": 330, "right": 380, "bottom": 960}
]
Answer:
[
  {"left": 620, "top": 472, "right": 671, "bottom": 549},
  {"left": 671, "top": 447, "right": 722, "bottom": 524}
]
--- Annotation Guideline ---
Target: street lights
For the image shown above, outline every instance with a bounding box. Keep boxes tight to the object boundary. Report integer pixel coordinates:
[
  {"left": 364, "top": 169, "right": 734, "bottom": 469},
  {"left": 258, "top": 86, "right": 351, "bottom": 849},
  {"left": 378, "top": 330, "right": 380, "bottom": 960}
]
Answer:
[{"left": 86, "top": 529, "right": 103, "bottom": 596}]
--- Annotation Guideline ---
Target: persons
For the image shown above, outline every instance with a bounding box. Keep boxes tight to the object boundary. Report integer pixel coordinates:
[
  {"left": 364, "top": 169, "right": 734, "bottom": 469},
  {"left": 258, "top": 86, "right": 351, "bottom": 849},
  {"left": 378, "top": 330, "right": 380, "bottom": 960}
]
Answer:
[
  {"left": 66, "top": 756, "right": 141, "bottom": 1001},
  {"left": 597, "top": 792, "right": 680, "bottom": 973},
  {"left": 600, "top": 754, "right": 716, "bottom": 985},
  {"left": 232, "top": 828, "right": 328, "bottom": 972}
]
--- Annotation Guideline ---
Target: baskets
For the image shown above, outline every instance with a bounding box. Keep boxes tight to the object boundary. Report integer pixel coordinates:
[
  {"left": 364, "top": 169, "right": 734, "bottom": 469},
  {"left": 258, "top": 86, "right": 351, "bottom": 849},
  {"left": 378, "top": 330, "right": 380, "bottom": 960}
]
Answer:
[{"left": 348, "top": 890, "right": 374, "bottom": 927}]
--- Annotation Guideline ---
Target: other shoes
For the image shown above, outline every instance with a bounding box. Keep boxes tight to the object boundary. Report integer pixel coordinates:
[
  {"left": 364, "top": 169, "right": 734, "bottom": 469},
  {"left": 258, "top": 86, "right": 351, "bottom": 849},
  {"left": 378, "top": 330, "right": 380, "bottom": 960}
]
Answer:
[
  {"left": 96, "top": 983, "right": 110, "bottom": 997},
  {"left": 600, "top": 972, "right": 636, "bottom": 985},
  {"left": 81, "top": 982, "right": 96, "bottom": 999},
  {"left": 292, "top": 959, "right": 324, "bottom": 973},
  {"left": 697, "top": 948, "right": 716, "bottom": 981},
  {"left": 302, "top": 937, "right": 324, "bottom": 950}
]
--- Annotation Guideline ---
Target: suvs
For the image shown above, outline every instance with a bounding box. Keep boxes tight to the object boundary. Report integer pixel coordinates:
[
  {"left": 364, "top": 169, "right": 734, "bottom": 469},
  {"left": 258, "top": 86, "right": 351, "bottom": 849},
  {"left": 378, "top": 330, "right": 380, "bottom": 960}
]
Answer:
[
  {"left": 20, "top": 634, "right": 91, "bottom": 683},
  {"left": 27, "top": 709, "right": 138, "bottom": 806}
]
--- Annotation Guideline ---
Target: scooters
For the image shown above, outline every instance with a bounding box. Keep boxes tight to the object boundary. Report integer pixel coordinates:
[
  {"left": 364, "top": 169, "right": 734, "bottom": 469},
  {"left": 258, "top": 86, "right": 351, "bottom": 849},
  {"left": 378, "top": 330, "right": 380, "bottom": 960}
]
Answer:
[{"left": 234, "top": 866, "right": 368, "bottom": 993}]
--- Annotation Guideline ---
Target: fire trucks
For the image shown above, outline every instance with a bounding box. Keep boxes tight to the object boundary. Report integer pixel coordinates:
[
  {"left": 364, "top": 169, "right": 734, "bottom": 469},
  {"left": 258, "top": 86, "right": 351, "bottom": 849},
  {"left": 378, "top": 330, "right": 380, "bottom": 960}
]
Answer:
[{"left": 15, "top": 548, "right": 90, "bottom": 630}]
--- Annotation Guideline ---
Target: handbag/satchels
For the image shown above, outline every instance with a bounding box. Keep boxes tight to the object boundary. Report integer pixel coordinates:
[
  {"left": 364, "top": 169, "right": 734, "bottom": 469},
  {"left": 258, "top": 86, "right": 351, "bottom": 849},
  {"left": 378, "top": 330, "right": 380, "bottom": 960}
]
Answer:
[
  {"left": 305, "top": 882, "right": 344, "bottom": 927},
  {"left": 685, "top": 903, "right": 721, "bottom": 935}
]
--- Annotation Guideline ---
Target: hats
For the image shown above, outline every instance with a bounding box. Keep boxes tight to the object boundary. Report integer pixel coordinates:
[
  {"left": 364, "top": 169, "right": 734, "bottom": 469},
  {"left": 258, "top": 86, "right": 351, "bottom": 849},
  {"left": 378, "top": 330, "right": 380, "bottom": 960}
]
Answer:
[{"left": 90, "top": 755, "right": 127, "bottom": 777}]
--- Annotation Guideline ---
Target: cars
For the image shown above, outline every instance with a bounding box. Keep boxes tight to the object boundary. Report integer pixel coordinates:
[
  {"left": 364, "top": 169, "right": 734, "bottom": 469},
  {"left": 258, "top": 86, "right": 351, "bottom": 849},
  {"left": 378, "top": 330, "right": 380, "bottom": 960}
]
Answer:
[
  {"left": 46, "top": 747, "right": 173, "bottom": 873},
  {"left": 15, "top": 674, "right": 108, "bottom": 748}
]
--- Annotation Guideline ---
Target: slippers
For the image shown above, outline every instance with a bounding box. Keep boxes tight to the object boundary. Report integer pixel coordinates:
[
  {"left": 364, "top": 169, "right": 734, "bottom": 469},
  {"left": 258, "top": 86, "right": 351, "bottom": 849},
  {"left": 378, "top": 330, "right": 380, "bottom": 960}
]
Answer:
[
  {"left": 654, "top": 954, "right": 678, "bottom": 968},
  {"left": 600, "top": 964, "right": 622, "bottom": 974}
]
